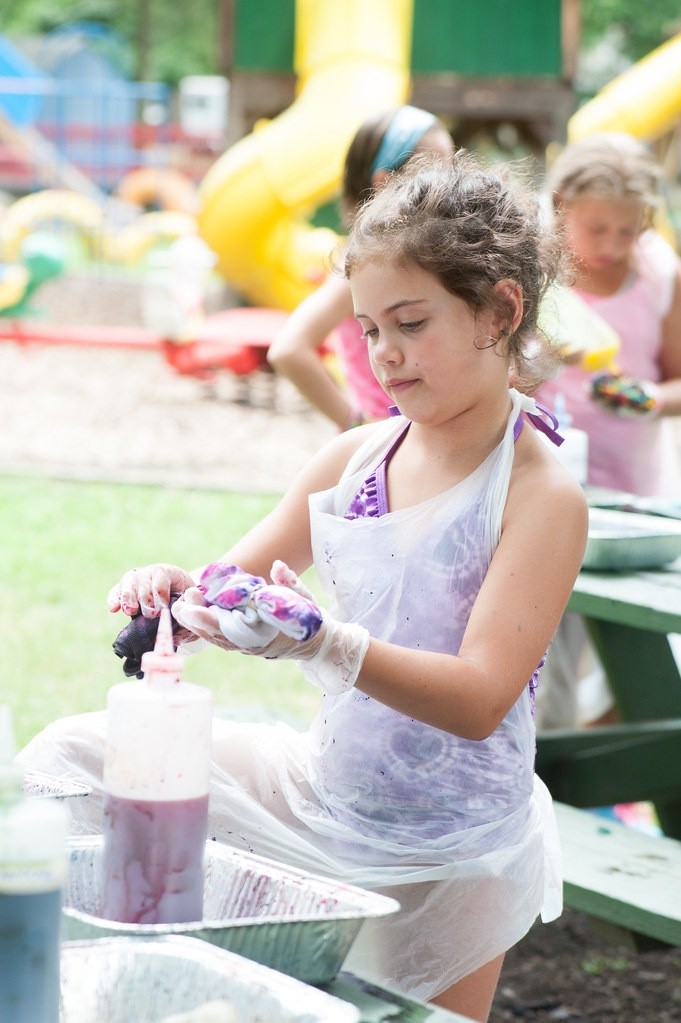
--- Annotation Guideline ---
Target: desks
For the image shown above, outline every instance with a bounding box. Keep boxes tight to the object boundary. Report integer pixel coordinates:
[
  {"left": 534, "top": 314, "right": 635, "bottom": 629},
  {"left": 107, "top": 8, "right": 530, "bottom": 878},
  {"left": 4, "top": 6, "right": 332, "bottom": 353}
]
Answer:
[{"left": 560, "top": 486, "right": 681, "bottom": 841}]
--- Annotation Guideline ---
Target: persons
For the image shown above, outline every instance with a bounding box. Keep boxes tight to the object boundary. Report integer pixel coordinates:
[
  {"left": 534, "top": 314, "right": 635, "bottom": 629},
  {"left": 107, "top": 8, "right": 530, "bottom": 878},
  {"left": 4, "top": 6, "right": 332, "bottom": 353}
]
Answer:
[
  {"left": 0, "top": 165, "right": 588, "bottom": 1023},
  {"left": 265, "top": 103, "right": 455, "bottom": 436},
  {"left": 516, "top": 134, "right": 681, "bottom": 500}
]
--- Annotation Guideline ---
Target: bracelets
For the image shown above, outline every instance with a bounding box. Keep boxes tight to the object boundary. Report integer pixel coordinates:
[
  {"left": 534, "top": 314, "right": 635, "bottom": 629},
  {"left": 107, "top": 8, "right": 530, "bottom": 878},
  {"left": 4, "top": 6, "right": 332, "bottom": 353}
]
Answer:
[{"left": 342, "top": 409, "right": 362, "bottom": 431}]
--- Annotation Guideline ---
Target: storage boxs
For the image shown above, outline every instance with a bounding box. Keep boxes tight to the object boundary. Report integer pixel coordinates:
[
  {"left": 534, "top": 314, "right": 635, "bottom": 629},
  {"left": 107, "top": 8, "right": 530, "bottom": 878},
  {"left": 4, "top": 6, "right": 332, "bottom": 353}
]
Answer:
[{"left": 55, "top": 834, "right": 403, "bottom": 1023}]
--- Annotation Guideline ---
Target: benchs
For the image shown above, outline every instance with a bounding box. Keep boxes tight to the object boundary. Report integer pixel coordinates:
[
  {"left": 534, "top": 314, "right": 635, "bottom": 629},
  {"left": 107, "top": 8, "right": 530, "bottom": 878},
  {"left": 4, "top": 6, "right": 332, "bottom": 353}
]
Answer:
[{"left": 554, "top": 801, "right": 681, "bottom": 948}]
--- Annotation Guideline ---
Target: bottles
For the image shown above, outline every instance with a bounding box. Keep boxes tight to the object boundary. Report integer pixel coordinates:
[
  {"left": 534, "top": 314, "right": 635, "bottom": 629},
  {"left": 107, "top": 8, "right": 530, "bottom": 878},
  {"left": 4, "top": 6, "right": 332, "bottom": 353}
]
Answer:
[
  {"left": 537, "top": 272, "right": 622, "bottom": 378},
  {"left": 101, "top": 609, "right": 211, "bottom": 925},
  {"left": 0, "top": 706, "right": 68, "bottom": 1023},
  {"left": 549, "top": 389, "right": 589, "bottom": 495}
]
